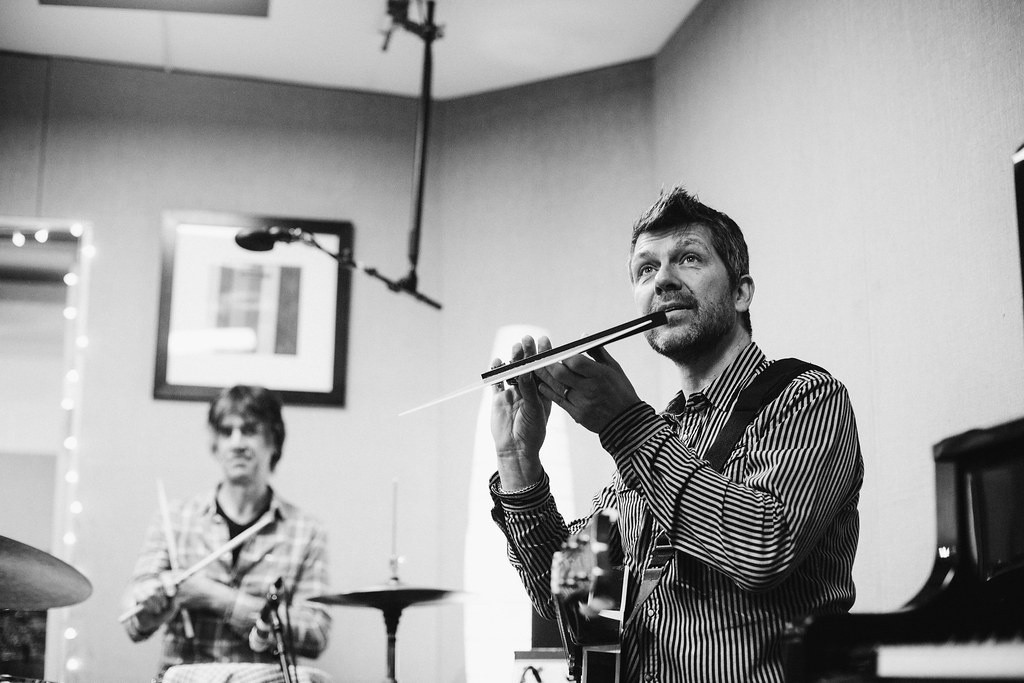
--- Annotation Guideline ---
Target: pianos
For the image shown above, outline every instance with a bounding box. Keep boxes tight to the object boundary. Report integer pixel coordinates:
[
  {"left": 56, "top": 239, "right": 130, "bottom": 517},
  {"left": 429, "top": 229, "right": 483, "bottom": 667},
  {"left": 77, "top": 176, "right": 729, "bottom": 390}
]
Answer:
[{"left": 562, "top": 414, "right": 1022, "bottom": 683}]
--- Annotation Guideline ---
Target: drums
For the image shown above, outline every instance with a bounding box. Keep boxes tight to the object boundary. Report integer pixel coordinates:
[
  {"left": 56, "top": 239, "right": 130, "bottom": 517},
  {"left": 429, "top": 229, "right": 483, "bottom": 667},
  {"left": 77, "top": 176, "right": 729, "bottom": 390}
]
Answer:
[{"left": 162, "top": 661, "right": 329, "bottom": 683}]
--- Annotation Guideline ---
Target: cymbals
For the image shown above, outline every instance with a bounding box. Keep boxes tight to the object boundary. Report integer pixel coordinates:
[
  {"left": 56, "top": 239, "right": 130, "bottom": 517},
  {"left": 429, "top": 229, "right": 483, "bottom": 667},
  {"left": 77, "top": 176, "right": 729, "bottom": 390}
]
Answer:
[
  {"left": 0, "top": 533, "right": 92, "bottom": 614},
  {"left": 307, "top": 585, "right": 481, "bottom": 608}
]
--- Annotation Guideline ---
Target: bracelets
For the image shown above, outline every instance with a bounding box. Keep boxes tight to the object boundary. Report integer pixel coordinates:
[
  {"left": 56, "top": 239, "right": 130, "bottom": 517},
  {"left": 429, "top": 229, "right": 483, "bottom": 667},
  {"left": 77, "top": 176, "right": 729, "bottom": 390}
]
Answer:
[{"left": 497, "top": 482, "right": 540, "bottom": 493}]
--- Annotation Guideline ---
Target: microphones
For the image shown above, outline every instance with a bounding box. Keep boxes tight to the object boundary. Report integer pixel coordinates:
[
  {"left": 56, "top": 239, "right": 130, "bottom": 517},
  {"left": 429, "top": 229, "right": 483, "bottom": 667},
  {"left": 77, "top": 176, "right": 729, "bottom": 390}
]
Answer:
[
  {"left": 249, "top": 577, "right": 285, "bottom": 653},
  {"left": 236, "top": 226, "right": 304, "bottom": 252}
]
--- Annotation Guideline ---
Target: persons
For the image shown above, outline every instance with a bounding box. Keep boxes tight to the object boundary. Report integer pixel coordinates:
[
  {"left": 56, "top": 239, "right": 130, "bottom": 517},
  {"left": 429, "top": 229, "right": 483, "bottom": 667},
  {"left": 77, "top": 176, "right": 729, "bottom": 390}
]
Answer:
[
  {"left": 120, "top": 385, "right": 335, "bottom": 682},
  {"left": 485, "top": 184, "right": 865, "bottom": 683}
]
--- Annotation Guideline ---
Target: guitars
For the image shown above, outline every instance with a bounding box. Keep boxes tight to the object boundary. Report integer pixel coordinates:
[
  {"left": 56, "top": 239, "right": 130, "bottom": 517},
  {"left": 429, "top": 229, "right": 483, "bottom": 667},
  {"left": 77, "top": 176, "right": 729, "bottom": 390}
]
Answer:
[{"left": 552, "top": 508, "right": 629, "bottom": 683}]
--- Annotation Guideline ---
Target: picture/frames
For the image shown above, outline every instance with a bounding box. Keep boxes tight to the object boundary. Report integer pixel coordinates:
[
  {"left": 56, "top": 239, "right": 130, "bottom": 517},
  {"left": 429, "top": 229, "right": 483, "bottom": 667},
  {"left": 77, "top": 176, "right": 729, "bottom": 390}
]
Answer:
[{"left": 152, "top": 209, "right": 356, "bottom": 409}]
